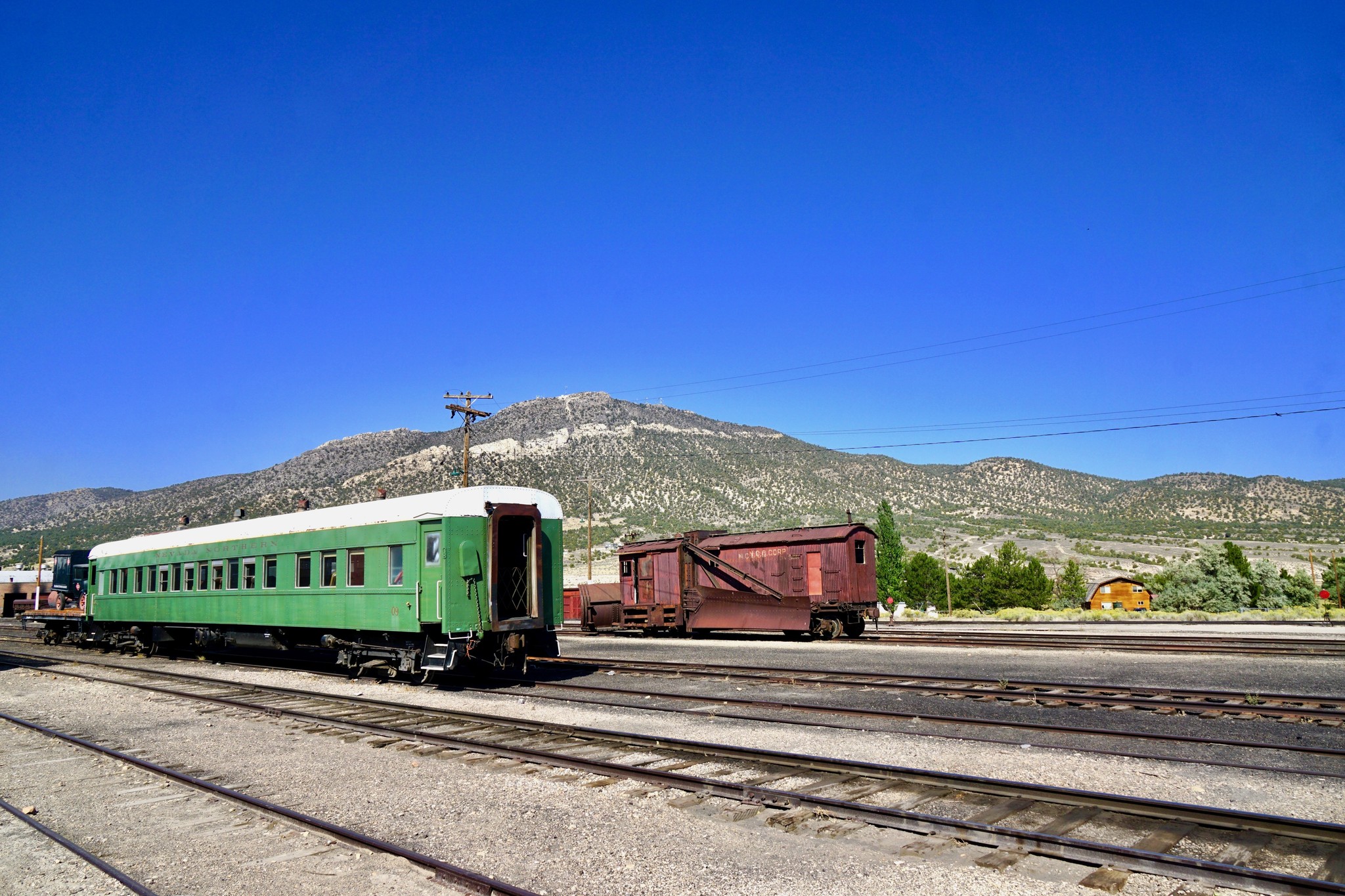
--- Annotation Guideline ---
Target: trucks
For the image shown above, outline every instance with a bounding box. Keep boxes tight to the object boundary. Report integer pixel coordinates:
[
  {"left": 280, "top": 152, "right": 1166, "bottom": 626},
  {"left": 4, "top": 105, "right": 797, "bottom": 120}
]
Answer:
[{"left": 51, "top": 549, "right": 92, "bottom": 611}]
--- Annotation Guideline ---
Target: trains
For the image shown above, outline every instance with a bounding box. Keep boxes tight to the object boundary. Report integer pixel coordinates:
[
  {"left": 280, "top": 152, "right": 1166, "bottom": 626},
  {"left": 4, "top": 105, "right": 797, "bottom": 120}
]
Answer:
[
  {"left": 24, "top": 485, "right": 568, "bottom": 687},
  {"left": 578, "top": 507, "right": 879, "bottom": 640}
]
